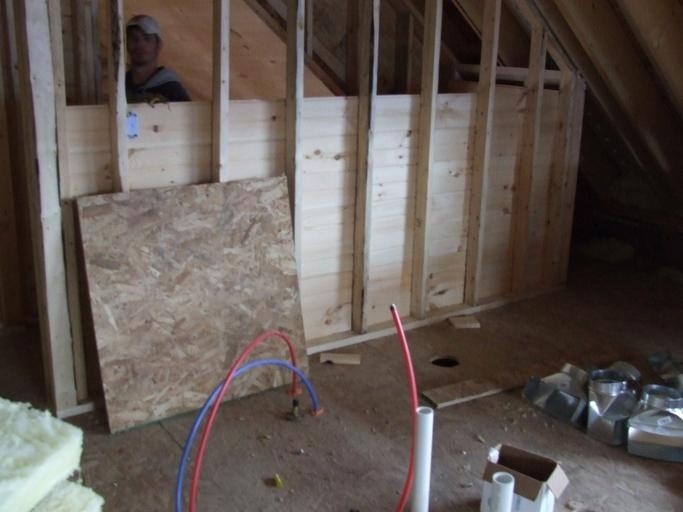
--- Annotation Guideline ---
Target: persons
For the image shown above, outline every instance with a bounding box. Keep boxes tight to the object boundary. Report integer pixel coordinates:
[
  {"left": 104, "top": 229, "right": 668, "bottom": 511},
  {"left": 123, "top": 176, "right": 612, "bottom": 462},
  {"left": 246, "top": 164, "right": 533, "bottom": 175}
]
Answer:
[{"left": 122, "top": 15, "right": 191, "bottom": 103}]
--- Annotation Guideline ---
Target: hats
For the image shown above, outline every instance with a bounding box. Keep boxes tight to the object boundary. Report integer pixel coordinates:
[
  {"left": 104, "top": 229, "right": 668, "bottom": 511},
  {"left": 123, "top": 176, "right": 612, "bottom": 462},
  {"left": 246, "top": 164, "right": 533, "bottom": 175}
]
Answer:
[{"left": 126, "top": 13, "right": 163, "bottom": 41}]
